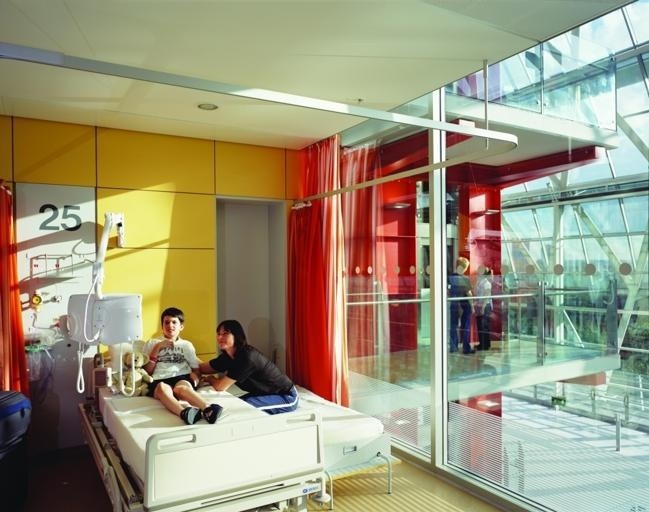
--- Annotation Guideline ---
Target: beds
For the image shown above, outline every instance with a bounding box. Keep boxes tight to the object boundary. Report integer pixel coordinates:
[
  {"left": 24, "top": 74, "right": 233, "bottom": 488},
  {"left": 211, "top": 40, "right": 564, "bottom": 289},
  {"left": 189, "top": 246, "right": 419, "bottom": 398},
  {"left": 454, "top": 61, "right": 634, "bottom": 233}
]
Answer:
[
  {"left": 78, "top": 348, "right": 327, "bottom": 512},
  {"left": 224, "top": 383, "right": 394, "bottom": 512}
]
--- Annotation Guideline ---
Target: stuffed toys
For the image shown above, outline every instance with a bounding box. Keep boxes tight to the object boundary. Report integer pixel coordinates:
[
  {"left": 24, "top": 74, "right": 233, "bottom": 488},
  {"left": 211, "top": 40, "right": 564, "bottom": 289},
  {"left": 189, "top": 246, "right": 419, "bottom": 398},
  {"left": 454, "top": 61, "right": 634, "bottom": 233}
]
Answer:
[{"left": 107, "top": 351, "right": 154, "bottom": 397}]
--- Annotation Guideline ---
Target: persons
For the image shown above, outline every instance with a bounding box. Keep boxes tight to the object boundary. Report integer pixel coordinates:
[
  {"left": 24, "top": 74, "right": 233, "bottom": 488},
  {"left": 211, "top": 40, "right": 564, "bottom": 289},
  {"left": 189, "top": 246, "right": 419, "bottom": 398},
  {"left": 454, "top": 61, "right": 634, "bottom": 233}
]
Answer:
[
  {"left": 143, "top": 307, "right": 224, "bottom": 425},
  {"left": 447, "top": 257, "right": 478, "bottom": 354},
  {"left": 198, "top": 318, "right": 300, "bottom": 415},
  {"left": 472, "top": 265, "right": 495, "bottom": 350}
]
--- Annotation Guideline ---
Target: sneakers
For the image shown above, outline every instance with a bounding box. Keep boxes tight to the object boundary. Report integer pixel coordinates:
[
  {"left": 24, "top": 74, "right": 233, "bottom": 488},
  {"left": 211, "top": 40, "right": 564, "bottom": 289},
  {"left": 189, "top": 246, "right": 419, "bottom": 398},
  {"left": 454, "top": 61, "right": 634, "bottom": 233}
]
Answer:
[
  {"left": 203, "top": 404, "right": 222, "bottom": 424},
  {"left": 450, "top": 342, "right": 490, "bottom": 354},
  {"left": 180, "top": 407, "right": 201, "bottom": 425}
]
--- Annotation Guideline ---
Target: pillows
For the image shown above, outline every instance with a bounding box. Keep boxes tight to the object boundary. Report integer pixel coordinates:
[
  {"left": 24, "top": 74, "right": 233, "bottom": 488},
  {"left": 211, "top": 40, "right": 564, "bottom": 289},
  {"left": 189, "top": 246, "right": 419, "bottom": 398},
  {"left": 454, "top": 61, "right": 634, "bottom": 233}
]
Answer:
[{"left": 108, "top": 341, "right": 144, "bottom": 384}]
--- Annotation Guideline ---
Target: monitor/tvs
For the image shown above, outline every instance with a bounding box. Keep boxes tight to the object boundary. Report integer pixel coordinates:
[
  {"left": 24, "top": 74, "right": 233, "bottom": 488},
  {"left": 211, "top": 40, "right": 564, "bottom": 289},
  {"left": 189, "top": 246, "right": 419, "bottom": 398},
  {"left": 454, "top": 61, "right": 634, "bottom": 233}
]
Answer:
[{"left": 67, "top": 294, "right": 142, "bottom": 344}]
